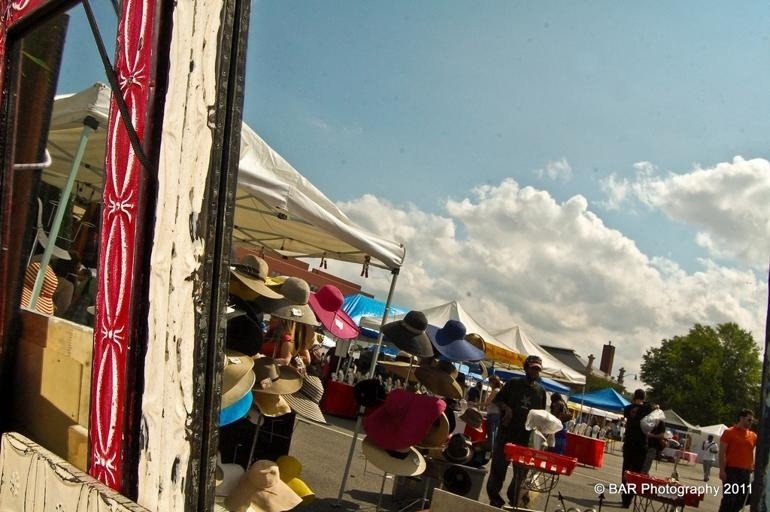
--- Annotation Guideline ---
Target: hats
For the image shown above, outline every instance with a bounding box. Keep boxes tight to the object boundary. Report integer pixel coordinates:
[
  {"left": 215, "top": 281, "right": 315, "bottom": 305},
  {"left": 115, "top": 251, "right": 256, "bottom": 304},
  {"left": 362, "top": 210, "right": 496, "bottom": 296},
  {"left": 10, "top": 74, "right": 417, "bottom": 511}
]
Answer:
[
  {"left": 525, "top": 356, "right": 542, "bottom": 370},
  {"left": 20, "top": 263, "right": 59, "bottom": 316},
  {"left": 227, "top": 254, "right": 361, "bottom": 339},
  {"left": 362, "top": 310, "right": 486, "bottom": 477},
  {"left": 219, "top": 351, "right": 329, "bottom": 428},
  {"left": 224, "top": 455, "right": 316, "bottom": 511}
]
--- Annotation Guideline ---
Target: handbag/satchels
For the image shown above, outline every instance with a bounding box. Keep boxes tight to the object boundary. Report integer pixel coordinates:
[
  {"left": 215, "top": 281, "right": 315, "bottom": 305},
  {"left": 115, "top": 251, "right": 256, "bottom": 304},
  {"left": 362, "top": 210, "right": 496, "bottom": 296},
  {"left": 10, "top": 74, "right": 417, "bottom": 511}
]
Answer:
[{"left": 559, "top": 409, "right": 572, "bottom": 423}]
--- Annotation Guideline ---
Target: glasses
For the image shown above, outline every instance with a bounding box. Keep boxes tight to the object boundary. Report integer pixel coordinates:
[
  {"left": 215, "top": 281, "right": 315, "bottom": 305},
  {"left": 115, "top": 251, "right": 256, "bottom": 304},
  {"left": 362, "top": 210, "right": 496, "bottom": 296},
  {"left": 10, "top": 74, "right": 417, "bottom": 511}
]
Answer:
[{"left": 748, "top": 419, "right": 752, "bottom": 421}]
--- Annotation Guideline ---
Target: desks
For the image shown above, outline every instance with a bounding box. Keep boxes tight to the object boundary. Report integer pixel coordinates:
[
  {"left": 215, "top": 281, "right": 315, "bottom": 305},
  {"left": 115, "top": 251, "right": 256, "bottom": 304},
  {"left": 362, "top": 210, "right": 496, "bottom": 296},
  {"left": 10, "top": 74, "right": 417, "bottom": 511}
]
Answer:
[
  {"left": 562, "top": 431, "right": 607, "bottom": 470},
  {"left": 321, "top": 381, "right": 383, "bottom": 421},
  {"left": 661, "top": 447, "right": 698, "bottom": 466}
]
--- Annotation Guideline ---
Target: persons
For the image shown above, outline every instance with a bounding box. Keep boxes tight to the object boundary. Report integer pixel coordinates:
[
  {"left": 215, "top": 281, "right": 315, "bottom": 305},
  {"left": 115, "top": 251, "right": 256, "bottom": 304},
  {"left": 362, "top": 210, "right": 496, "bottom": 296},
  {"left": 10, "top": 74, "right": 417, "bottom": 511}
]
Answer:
[
  {"left": 642, "top": 399, "right": 667, "bottom": 477},
  {"left": 718, "top": 408, "right": 758, "bottom": 511},
  {"left": 569, "top": 409, "right": 627, "bottom": 442},
  {"left": 549, "top": 392, "right": 569, "bottom": 454},
  {"left": 701, "top": 434, "right": 719, "bottom": 482},
  {"left": 262, "top": 312, "right": 296, "bottom": 368},
  {"left": 486, "top": 354, "right": 548, "bottom": 509},
  {"left": 466, "top": 374, "right": 503, "bottom": 459},
  {"left": 622, "top": 388, "right": 653, "bottom": 509},
  {"left": 225, "top": 303, "right": 265, "bottom": 360},
  {"left": 299, "top": 340, "right": 419, "bottom": 391},
  {"left": 465, "top": 381, "right": 482, "bottom": 404},
  {"left": 664, "top": 429, "right": 692, "bottom": 451}
]
